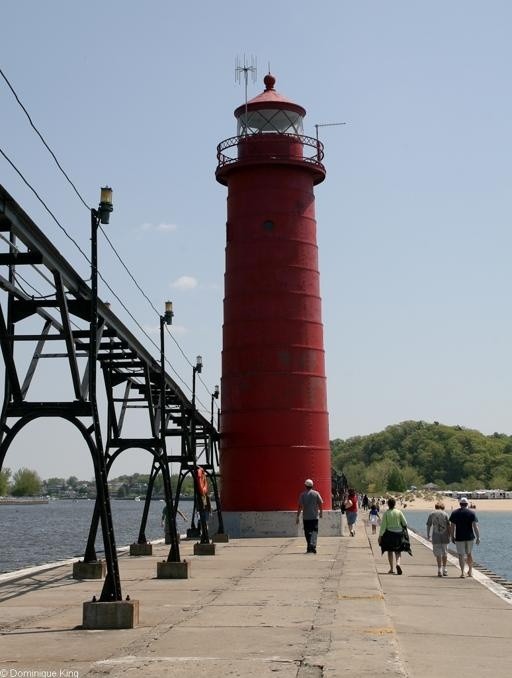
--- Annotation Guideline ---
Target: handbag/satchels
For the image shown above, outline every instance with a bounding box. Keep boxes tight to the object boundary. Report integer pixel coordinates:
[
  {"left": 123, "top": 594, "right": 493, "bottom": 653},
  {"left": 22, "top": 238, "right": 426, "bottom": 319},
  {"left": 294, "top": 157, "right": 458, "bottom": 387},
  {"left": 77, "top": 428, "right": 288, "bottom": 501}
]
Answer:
[{"left": 296, "top": 517, "right": 299, "bottom": 518}]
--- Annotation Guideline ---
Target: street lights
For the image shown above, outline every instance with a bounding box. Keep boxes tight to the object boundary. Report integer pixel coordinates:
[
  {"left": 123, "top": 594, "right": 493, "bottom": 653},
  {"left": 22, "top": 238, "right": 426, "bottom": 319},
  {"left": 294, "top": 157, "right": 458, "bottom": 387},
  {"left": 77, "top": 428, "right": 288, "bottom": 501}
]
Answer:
[{"left": 343, "top": 498, "right": 353, "bottom": 509}]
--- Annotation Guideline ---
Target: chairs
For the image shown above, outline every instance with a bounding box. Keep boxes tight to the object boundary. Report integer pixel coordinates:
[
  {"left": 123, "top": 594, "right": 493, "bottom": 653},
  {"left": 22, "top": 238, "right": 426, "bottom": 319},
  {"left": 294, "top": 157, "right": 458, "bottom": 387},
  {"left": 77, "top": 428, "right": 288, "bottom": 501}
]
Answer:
[
  {"left": 438, "top": 571, "right": 443, "bottom": 576},
  {"left": 309, "top": 542, "right": 316, "bottom": 552},
  {"left": 350, "top": 528, "right": 354, "bottom": 536},
  {"left": 443, "top": 569, "right": 449, "bottom": 575}
]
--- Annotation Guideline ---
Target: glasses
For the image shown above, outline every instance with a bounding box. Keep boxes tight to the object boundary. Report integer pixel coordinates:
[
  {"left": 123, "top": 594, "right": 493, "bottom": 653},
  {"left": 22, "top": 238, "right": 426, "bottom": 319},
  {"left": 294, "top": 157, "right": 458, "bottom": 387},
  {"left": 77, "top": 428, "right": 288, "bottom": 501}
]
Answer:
[
  {"left": 215, "top": 384, "right": 219, "bottom": 399},
  {"left": 196, "top": 354, "right": 203, "bottom": 373},
  {"left": 164, "top": 300, "right": 174, "bottom": 325},
  {"left": 99, "top": 185, "right": 113, "bottom": 224}
]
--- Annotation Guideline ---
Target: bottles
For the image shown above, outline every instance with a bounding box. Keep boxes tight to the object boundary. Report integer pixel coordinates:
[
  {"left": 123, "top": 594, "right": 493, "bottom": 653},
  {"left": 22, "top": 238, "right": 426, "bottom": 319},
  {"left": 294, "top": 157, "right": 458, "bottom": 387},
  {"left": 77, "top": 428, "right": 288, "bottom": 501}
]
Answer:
[
  {"left": 460, "top": 497, "right": 469, "bottom": 503},
  {"left": 304, "top": 478, "right": 313, "bottom": 486}
]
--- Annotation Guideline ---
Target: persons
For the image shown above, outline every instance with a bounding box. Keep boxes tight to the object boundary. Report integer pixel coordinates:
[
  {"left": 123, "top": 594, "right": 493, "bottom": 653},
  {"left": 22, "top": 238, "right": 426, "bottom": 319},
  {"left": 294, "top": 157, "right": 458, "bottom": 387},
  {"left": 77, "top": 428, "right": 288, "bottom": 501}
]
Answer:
[
  {"left": 334, "top": 488, "right": 385, "bottom": 536},
  {"left": 295, "top": 479, "right": 324, "bottom": 553},
  {"left": 380, "top": 500, "right": 407, "bottom": 575},
  {"left": 160, "top": 501, "right": 188, "bottom": 532},
  {"left": 427, "top": 503, "right": 452, "bottom": 578},
  {"left": 450, "top": 498, "right": 480, "bottom": 579}
]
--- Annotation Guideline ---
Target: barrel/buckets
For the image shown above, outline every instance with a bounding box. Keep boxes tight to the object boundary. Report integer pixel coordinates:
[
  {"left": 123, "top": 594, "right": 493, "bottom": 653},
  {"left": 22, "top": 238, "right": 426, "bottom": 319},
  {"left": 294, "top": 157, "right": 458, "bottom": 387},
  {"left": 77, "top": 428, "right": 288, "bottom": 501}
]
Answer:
[
  {"left": 396, "top": 564, "right": 402, "bottom": 574},
  {"left": 389, "top": 570, "right": 395, "bottom": 573}
]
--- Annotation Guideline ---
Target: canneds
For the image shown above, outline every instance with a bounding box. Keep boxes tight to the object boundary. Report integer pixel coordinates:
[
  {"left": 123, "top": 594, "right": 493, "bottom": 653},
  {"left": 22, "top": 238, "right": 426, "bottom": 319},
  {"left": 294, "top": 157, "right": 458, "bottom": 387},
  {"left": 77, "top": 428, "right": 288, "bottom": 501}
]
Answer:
[{"left": 197, "top": 465, "right": 208, "bottom": 496}]
